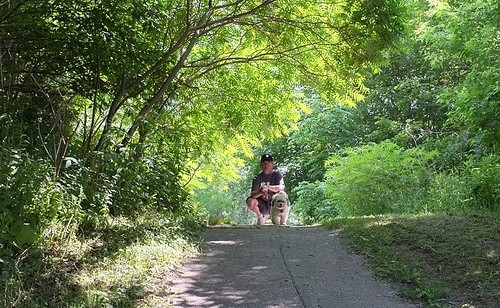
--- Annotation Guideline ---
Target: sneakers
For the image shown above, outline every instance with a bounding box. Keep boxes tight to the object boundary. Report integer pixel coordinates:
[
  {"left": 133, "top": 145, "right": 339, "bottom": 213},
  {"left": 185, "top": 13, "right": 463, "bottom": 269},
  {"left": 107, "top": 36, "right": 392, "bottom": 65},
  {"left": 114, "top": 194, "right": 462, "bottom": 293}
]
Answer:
[{"left": 257, "top": 216, "right": 266, "bottom": 225}]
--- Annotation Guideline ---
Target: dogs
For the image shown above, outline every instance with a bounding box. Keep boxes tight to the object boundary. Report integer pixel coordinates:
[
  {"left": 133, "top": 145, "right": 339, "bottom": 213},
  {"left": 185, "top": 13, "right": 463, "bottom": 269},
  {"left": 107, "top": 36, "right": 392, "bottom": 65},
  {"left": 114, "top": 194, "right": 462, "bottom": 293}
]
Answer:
[{"left": 270, "top": 191, "right": 290, "bottom": 225}]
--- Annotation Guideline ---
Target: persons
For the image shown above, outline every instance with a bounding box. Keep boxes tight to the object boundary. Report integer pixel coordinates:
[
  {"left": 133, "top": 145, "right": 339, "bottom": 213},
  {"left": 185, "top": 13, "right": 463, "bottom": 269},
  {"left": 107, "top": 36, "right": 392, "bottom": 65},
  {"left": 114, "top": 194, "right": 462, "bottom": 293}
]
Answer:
[{"left": 246, "top": 154, "right": 285, "bottom": 224}]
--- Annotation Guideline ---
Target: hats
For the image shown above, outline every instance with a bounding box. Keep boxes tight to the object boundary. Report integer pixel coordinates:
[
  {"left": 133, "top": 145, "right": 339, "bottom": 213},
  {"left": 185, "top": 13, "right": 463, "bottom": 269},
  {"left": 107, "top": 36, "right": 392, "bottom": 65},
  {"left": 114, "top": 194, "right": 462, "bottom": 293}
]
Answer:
[{"left": 261, "top": 154, "right": 273, "bottom": 162}]
